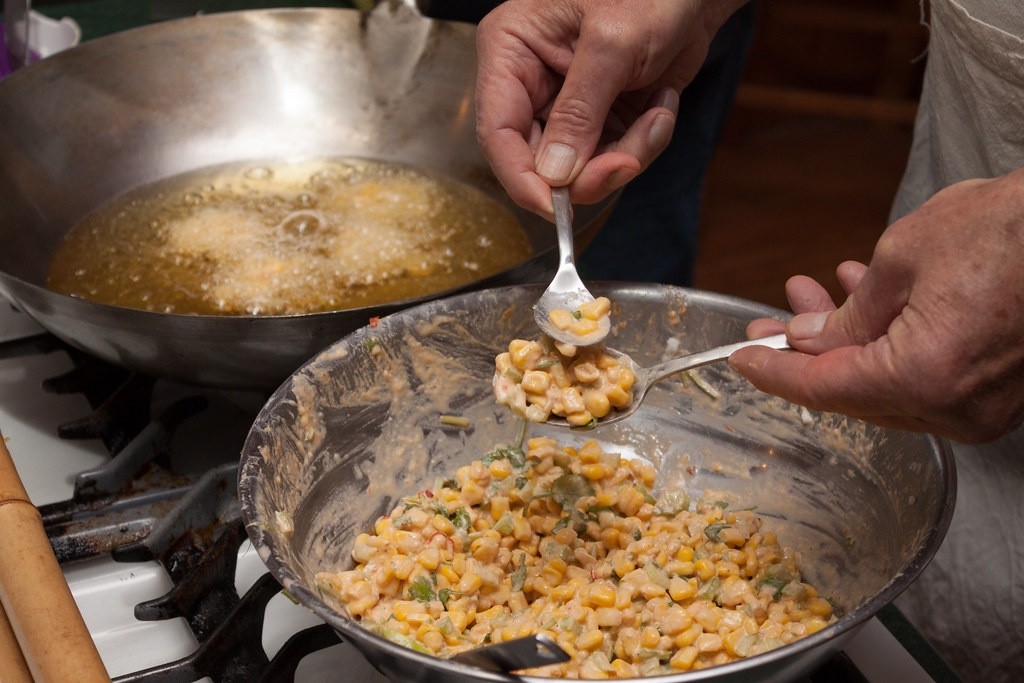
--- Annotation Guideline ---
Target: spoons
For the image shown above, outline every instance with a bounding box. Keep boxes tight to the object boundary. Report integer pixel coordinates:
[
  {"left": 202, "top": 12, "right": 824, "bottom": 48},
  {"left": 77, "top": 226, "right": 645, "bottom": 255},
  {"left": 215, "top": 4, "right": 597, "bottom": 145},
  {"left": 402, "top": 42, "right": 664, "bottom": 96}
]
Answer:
[
  {"left": 492, "top": 331, "right": 796, "bottom": 428},
  {"left": 532, "top": 187, "right": 611, "bottom": 346}
]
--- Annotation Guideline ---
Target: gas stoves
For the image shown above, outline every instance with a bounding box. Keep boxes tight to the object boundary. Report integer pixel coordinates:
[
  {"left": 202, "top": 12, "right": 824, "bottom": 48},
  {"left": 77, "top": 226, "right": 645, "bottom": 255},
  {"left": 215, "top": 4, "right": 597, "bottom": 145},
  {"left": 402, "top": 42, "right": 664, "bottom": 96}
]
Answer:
[{"left": 10, "top": 327, "right": 392, "bottom": 683}]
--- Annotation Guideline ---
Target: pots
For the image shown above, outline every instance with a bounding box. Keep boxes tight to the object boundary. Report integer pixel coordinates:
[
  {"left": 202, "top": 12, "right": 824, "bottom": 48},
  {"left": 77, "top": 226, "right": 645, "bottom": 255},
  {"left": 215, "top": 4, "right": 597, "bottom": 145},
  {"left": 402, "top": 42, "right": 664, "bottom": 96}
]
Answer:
[{"left": 0, "top": 0, "right": 633, "bottom": 396}]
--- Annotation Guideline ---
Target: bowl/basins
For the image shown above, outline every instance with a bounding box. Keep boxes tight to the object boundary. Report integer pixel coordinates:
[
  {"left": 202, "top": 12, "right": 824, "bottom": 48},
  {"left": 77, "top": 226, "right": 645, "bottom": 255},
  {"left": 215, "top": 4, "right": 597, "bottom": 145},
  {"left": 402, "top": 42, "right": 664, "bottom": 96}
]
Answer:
[{"left": 241, "top": 278, "right": 958, "bottom": 683}]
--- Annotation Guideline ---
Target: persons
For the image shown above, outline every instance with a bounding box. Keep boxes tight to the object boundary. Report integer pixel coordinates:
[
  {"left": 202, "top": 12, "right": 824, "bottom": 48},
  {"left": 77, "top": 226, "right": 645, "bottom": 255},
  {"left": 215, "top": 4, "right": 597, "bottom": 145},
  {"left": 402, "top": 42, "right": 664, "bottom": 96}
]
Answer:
[{"left": 475, "top": 0, "right": 1024, "bottom": 683}]
